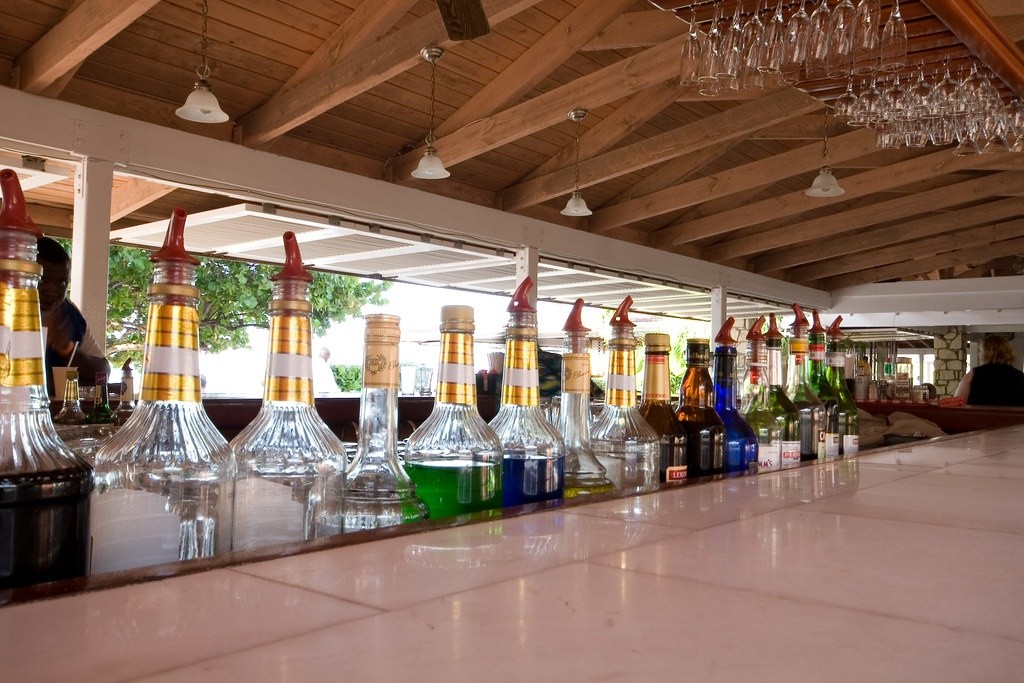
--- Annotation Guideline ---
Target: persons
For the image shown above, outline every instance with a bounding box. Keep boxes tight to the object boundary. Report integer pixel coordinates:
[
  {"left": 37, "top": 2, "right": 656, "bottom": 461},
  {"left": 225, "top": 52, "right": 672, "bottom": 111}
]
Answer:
[
  {"left": 312, "top": 348, "right": 340, "bottom": 392},
  {"left": 537, "top": 343, "right": 603, "bottom": 398},
  {"left": 952, "top": 335, "right": 1024, "bottom": 408},
  {"left": 35, "top": 234, "right": 110, "bottom": 392}
]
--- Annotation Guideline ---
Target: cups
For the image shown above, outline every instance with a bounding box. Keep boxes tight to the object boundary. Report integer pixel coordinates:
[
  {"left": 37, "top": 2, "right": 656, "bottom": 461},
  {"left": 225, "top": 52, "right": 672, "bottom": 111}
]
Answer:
[
  {"left": 420, "top": 368, "right": 433, "bottom": 394},
  {"left": 52, "top": 367, "right": 78, "bottom": 400},
  {"left": 540, "top": 396, "right": 605, "bottom": 433},
  {"left": 496, "top": 374, "right": 503, "bottom": 396},
  {"left": 401, "top": 366, "right": 416, "bottom": 395}
]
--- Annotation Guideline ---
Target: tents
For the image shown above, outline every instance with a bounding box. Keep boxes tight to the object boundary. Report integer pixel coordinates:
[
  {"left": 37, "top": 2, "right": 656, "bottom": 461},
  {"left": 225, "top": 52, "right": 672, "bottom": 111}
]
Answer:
[{"left": 411, "top": 306, "right": 605, "bottom": 351}]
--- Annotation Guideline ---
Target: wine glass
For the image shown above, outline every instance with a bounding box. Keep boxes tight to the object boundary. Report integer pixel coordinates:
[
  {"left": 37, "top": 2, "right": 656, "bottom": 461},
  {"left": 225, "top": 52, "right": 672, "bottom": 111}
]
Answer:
[{"left": 679, "top": 0, "right": 1024, "bottom": 156}]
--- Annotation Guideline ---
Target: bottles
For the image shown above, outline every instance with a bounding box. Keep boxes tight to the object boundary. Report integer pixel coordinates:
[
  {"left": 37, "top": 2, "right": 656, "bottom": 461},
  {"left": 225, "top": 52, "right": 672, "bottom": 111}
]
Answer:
[
  {"left": 54, "top": 357, "right": 136, "bottom": 425},
  {"left": 405, "top": 305, "right": 503, "bottom": 520},
  {"left": 0, "top": 169, "right": 96, "bottom": 593},
  {"left": 826, "top": 316, "right": 859, "bottom": 454},
  {"left": 591, "top": 296, "right": 661, "bottom": 490},
  {"left": 553, "top": 298, "right": 616, "bottom": 499},
  {"left": 855, "top": 356, "right": 929, "bottom": 401},
  {"left": 89, "top": 208, "right": 235, "bottom": 575},
  {"left": 476, "top": 369, "right": 496, "bottom": 395},
  {"left": 486, "top": 276, "right": 565, "bottom": 507},
  {"left": 675, "top": 303, "right": 839, "bottom": 480},
  {"left": 637, "top": 333, "right": 688, "bottom": 484},
  {"left": 228, "top": 231, "right": 346, "bottom": 551},
  {"left": 343, "top": 314, "right": 429, "bottom": 533}
]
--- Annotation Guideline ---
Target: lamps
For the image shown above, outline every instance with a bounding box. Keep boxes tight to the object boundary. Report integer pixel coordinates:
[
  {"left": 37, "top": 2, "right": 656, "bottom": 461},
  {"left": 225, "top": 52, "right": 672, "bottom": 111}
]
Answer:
[
  {"left": 175, "top": 0, "right": 231, "bottom": 125},
  {"left": 807, "top": 107, "right": 846, "bottom": 197},
  {"left": 411, "top": 46, "right": 452, "bottom": 181},
  {"left": 560, "top": 108, "right": 593, "bottom": 218}
]
